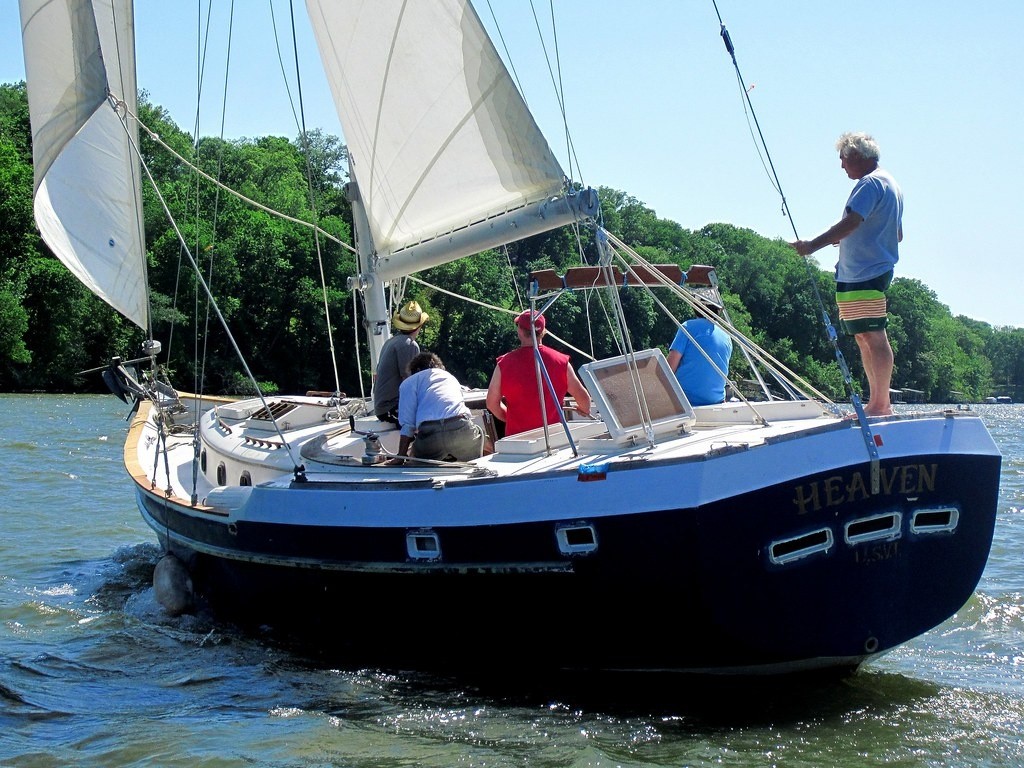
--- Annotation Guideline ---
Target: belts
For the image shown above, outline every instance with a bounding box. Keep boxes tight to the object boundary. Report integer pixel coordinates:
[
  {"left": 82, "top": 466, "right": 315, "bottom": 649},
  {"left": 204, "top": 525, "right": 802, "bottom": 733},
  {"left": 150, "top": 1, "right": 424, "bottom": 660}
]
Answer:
[{"left": 419, "top": 415, "right": 466, "bottom": 426}]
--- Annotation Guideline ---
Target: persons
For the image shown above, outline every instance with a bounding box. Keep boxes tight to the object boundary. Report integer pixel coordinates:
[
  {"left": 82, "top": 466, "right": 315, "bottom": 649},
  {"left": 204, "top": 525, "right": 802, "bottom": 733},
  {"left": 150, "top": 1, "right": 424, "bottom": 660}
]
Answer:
[
  {"left": 486, "top": 309, "right": 592, "bottom": 436},
  {"left": 386, "top": 352, "right": 484, "bottom": 466},
  {"left": 792, "top": 130, "right": 903, "bottom": 423},
  {"left": 667, "top": 294, "right": 733, "bottom": 407},
  {"left": 373, "top": 300, "right": 429, "bottom": 423}
]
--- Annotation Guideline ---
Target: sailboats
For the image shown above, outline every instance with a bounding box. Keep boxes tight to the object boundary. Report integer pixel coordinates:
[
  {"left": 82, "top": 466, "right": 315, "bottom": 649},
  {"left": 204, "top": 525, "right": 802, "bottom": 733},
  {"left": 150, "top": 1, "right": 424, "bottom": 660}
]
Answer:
[{"left": 17, "top": 0, "right": 1002, "bottom": 680}]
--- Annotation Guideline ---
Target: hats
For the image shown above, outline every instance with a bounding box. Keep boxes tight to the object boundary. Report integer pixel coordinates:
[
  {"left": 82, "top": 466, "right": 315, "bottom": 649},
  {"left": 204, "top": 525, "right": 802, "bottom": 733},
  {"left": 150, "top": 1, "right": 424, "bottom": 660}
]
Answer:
[
  {"left": 692, "top": 289, "right": 717, "bottom": 305},
  {"left": 514, "top": 309, "right": 545, "bottom": 334},
  {"left": 392, "top": 300, "right": 429, "bottom": 330}
]
